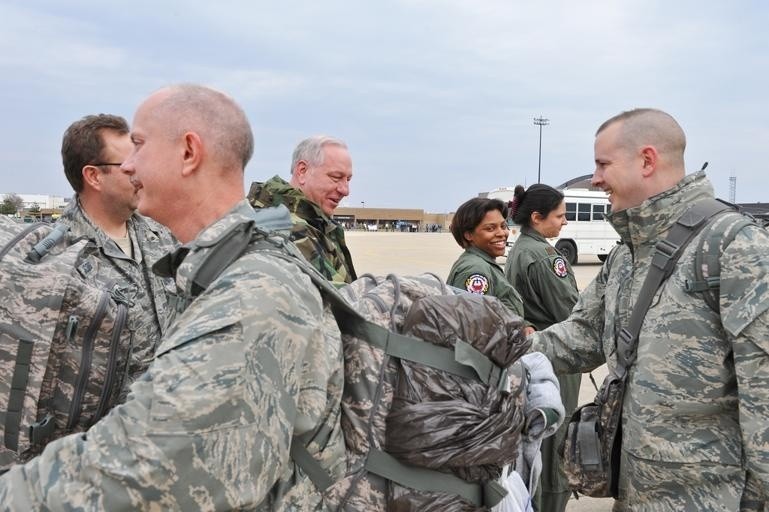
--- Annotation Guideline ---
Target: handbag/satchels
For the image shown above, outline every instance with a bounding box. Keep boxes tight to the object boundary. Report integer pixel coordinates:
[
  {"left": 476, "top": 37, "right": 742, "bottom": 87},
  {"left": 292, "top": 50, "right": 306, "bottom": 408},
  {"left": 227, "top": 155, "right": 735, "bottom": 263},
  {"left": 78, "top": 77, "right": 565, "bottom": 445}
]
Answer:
[{"left": 556, "top": 372, "right": 624, "bottom": 500}]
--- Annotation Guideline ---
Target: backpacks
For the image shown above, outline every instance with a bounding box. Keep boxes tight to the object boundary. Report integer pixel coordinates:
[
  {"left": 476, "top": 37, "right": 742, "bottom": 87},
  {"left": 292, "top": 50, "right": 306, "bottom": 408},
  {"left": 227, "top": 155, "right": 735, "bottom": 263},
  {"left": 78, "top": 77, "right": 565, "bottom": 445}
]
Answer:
[
  {"left": 0, "top": 215, "right": 136, "bottom": 466},
  {"left": 240, "top": 247, "right": 566, "bottom": 512}
]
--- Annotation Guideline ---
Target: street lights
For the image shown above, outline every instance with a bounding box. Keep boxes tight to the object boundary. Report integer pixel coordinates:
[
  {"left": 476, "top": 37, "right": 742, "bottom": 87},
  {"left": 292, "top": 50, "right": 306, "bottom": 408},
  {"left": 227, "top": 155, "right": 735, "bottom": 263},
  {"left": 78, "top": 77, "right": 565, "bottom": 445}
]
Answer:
[{"left": 533, "top": 115, "right": 549, "bottom": 184}]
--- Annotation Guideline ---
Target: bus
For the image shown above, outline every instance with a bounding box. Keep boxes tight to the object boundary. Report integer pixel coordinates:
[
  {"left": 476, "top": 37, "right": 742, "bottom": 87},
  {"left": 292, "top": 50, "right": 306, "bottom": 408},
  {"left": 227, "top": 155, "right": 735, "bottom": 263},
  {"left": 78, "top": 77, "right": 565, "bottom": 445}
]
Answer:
[{"left": 487, "top": 186, "right": 622, "bottom": 264}]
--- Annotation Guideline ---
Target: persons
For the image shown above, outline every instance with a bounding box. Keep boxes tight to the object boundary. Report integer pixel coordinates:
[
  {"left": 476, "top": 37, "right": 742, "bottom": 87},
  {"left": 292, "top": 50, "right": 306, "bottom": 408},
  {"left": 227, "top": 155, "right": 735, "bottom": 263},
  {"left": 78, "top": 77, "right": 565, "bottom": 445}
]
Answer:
[
  {"left": 0, "top": 81, "right": 359, "bottom": 512},
  {"left": 55, "top": 110, "right": 188, "bottom": 407},
  {"left": 337, "top": 220, "right": 441, "bottom": 232},
  {"left": 444, "top": 196, "right": 540, "bottom": 342},
  {"left": 505, "top": 182, "right": 584, "bottom": 512},
  {"left": 251, "top": 135, "right": 357, "bottom": 292},
  {"left": 522, "top": 108, "right": 769, "bottom": 512}
]
